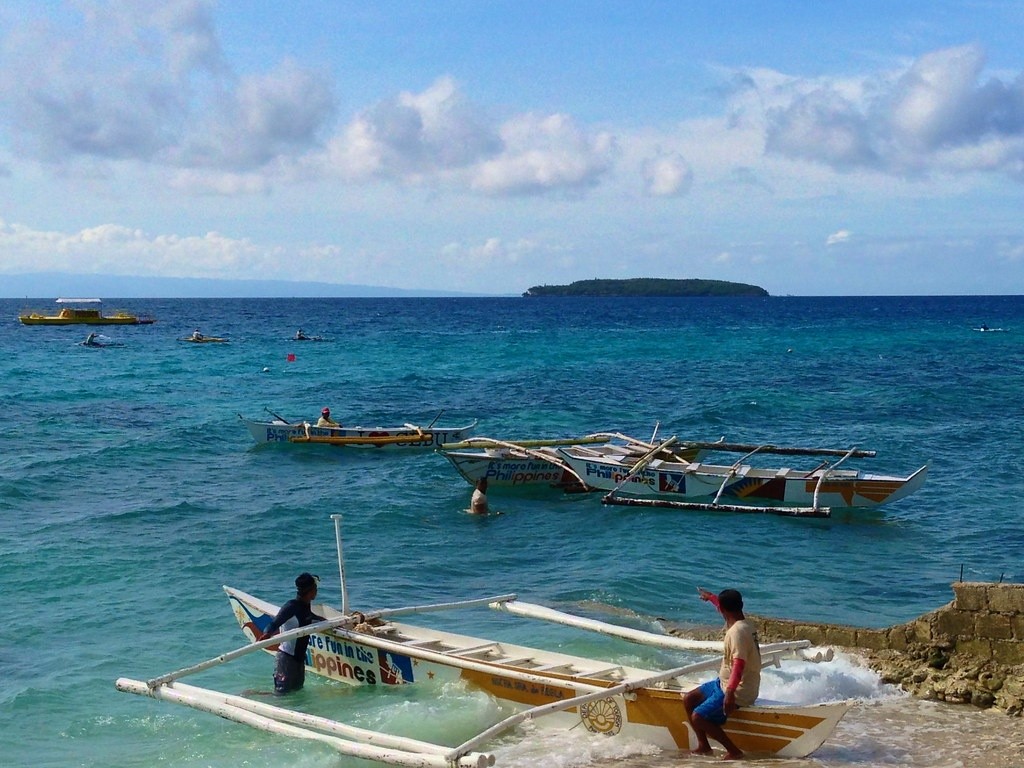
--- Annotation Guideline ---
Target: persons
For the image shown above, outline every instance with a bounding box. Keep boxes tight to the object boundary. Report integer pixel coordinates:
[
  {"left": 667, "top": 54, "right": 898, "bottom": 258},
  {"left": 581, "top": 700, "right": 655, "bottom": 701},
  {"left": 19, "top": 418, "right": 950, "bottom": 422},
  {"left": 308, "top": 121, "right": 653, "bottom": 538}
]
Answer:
[
  {"left": 470, "top": 477, "right": 488, "bottom": 515},
  {"left": 682, "top": 587, "right": 762, "bottom": 761},
  {"left": 317, "top": 407, "right": 343, "bottom": 428},
  {"left": 193, "top": 329, "right": 203, "bottom": 340},
  {"left": 297, "top": 329, "right": 305, "bottom": 339},
  {"left": 239, "top": 572, "right": 328, "bottom": 698},
  {"left": 87, "top": 332, "right": 98, "bottom": 345}
]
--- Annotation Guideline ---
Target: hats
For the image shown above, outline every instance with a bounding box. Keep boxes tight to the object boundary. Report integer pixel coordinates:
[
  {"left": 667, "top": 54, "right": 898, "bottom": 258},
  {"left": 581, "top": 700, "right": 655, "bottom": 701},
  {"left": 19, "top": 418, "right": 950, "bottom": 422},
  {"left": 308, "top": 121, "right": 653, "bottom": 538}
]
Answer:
[{"left": 322, "top": 407, "right": 330, "bottom": 413}]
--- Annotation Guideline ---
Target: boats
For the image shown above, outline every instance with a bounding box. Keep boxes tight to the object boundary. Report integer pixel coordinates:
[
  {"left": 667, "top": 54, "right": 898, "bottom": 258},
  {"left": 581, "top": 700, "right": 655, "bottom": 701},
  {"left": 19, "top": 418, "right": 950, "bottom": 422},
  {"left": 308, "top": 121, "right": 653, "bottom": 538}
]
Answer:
[
  {"left": 82, "top": 341, "right": 124, "bottom": 348},
  {"left": 554, "top": 445, "right": 931, "bottom": 511},
  {"left": 290, "top": 336, "right": 336, "bottom": 343},
  {"left": 434, "top": 436, "right": 727, "bottom": 491},
  {"left": 223, "top": 586, "right": 862, "bottom": 761},
  {"left": 237, "top": 411, "right": 481, "bottom": 445},
  {"left": 177, "top": 338, "right": 229, "bottom": 343},
  {"left": 17, "top": 298, "right": 155, "bottom": 326}
]
